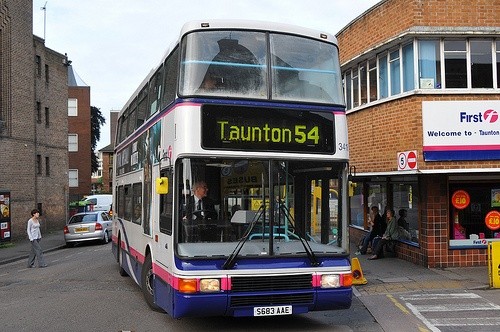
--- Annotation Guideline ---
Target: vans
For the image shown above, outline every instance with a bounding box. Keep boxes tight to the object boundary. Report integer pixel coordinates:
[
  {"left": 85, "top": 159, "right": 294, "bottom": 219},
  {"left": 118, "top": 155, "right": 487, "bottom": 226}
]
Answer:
[{"left": 84, "top": 193, "right": 112, "bottom": 210}]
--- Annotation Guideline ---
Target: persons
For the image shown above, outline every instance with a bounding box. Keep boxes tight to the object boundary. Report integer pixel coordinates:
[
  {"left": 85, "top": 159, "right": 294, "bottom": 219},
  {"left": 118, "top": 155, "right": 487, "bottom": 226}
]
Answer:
[
  {"left": 397, "top": 209, "right": 410, "bottom": 241},
  {"left": 27, "top": 209, "right": 48, "bottom": 268},
  {"left": 274, "top": 195, "right": 290, "bottom": 224},
  {"left": 180, "top": 180, "right": 218, "bottom": 221},
  {"left": 354, "top": 206, "right": 384, "bottom": 255},
  {"left": 367, "top": 208, "right": 399, "bottom": 260}
]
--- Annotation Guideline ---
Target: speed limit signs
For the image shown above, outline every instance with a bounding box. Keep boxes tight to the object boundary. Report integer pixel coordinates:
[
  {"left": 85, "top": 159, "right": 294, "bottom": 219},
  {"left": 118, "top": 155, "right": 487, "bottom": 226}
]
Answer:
[{"left": 398, "top": 153, "right": 407, "bottom": 170}]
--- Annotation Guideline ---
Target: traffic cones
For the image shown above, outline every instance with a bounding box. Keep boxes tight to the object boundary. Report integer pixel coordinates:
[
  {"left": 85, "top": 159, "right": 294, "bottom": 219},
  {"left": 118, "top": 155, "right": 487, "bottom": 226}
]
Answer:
[{"left": 351, "top": 257, "right": 367, "bottom": 285}]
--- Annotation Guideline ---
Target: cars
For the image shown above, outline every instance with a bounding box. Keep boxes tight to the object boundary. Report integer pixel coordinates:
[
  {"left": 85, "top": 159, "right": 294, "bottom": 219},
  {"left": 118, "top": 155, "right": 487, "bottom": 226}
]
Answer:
[{"left": 64, "top": 210, "right": 112, "bottom": 247}]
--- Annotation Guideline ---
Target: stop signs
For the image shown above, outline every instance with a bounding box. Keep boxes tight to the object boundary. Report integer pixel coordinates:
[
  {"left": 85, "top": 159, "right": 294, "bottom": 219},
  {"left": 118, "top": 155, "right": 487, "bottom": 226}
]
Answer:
[{"left": 407, "top": 151, "right": 416, "bottom": 168}]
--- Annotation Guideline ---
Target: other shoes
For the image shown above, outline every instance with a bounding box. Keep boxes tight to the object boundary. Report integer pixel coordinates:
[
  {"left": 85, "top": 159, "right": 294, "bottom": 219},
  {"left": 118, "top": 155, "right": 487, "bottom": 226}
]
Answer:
[
  {"left": 368, "top": 255, "right": 377, "bottom": 259},
  {"left": 28, "top": 266, "right": 36, "bottom": 268},
  {"left": 39, "top": 264, "right": 47, "bottom": 266}
]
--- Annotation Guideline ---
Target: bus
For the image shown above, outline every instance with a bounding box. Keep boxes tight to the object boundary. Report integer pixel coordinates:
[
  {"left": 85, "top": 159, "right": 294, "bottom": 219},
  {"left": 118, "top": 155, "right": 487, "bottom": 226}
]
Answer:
[{"left": 111, "top": 17, "right": 353, "bottom": 320}]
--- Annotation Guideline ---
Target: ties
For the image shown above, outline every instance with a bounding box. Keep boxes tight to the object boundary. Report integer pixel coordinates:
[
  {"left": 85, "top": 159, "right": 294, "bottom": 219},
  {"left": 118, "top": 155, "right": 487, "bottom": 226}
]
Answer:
[{"left": 197, "top": 200, "right": 202, "bottom": 219}]
